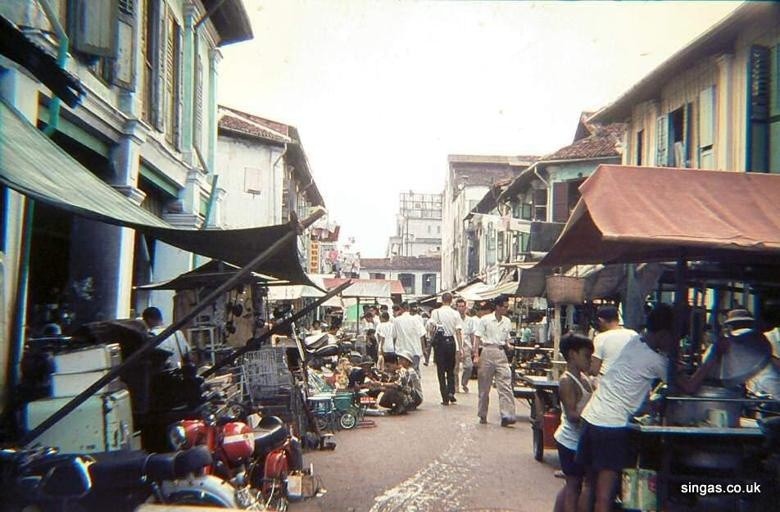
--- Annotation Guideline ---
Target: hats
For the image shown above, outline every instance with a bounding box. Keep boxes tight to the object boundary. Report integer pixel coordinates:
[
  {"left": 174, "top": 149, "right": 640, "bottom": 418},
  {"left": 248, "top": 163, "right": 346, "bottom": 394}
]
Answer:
[
  {"left": 392, "top": 305, "right": 400, "bottom": 311},
  {"left": 360, "top": 311, "right": 372, "bottom": 320},
  {"left": 409, "top": 302, "right": 418, "bottom": 307},
  {"left": 723, "top": 307, "right": 757, "bottom": 324},
  {"left": 395, "top": 350, "right": 415, "bottom": 365},
  {"left": 400, "top": 302, "right": 409, "bottom": 308}
]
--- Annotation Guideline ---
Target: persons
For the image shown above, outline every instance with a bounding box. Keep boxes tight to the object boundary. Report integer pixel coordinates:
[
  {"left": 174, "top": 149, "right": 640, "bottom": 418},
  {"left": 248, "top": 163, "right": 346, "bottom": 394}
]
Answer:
[
  {"left": 311, "top": 316, "right": 346, "bottom": 350},
  {"left": 410, "top": 304, "right": 430, "bottom": 325},
  {"left": 452, "top": 299, "right": 475, "bottom": 392},
  {"left": 397, "top": 352, "right": 423, "bottom": 411},
  {"left": 521, "top": 313, "right": 553, "bottom": 347},
  {"left": 471, "top": 295, "right": 518, "bottom": 427},
  {"left": 143, "top": 307, "right": 192, "bottom": 370},
  {"left": 375, "top": 352, "right": 415, "bottom": 414},
  {"left": 359, "top": 303, "right": 376, "bottom": 347},
  {"left": 554, "top": 335, "right": 597, "bottom": 512},
  {"left": 353, "top": 355, "right": 382, "bottom": 409},
  {"left": 574, "top": 306, "right": 729, "bottom": 512},
  {"left": 725, "top": 309, "right": 779, "bottom": 401},
  {"left": 591, "top": 307, "right": 639, "bottom": 376},
  {"left": 381, "top": 306, "right": 395, "bottom": 322},
  {"left": 469, "top": 309, "right": 480, "bottom": 379},
  {"left": 392, "top": 303, "right": 428, "bottom": 378},
  {"left": 429, "top": 293, "right": 463, "bottom": 404},
  {"left": 374, "top": 312, "right": 395, "bottom": 356}
]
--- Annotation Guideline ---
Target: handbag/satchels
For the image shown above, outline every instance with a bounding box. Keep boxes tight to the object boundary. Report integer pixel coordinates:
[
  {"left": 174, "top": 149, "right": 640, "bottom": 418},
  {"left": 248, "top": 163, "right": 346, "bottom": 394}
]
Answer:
[
  {"left": 429, "top": 309, "right": 444, "bottom": 347},
  {"left": 379, "top": 388, "right": 403, "bottom": 408},
  {"left": 174, "top": 330, "right": 186, "bottom": 363}
]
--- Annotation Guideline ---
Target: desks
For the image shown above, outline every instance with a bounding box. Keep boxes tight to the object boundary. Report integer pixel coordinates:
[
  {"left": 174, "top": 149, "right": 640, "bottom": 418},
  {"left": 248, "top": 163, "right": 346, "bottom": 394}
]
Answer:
[
  {"left": 524, "top": 375, "right": 597, "bottom": 461},
  {"left": 627, "top": 415, "right": 780, "bottom": 512},
  {"left": 515, "top": 347, "right": 566, "bottom": 375}
]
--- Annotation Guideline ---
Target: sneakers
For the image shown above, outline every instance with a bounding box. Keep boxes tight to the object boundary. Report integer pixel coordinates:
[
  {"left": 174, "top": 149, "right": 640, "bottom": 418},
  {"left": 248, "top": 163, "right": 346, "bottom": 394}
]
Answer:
[
  {"left": 462, "top": 386, "right": 469, "bottom": 392},
  {"left": 454, "top": 388, "right": 461, "bottom": 393}
]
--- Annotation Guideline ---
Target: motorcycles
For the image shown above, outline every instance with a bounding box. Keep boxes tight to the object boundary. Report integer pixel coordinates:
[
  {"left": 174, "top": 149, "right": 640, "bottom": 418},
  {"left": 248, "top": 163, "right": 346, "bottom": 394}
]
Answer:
[
  {"left": 5, "top": 440, "right": 265, "bottom": 512},
  {"left": 297, "top": 327, "right": 402, "bottom": 417},
  {"left": 158, "top": 395, "right": 306, "bottom": 510}
]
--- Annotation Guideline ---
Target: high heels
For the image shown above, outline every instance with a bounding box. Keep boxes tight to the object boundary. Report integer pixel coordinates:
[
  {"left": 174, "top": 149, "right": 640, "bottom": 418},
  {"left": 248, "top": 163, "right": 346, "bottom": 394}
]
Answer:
[
  {"left": 414, "top": 406, "right": 417, "bottom": 409},
  {"left": 387, "top": 409, "right": 392, "bottom": 413},
  {"left": 391, "top": 409, "right": 406, "bottom": 415}
]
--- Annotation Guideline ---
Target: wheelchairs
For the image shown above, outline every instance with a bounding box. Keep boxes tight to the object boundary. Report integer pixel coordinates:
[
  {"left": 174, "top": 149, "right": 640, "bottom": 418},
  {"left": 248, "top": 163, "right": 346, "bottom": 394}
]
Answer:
[{"left": 299, "top": 384, "right": 357, "bottom": 431}]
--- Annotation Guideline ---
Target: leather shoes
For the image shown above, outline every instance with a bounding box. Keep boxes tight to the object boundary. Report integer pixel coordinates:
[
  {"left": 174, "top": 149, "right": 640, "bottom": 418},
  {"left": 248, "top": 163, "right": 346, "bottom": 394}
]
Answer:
[
  {"left": 479, "top": 417, "right": 487, "bottom": 425},
  {"left": 448, "top": 396, "right": 456, "bottom": 402},
  {"left": 501, "top": 416, "right": 516, "bottom": 427},
  {"left": 440, "top": 402, "right": 449, "bottom": 405}
]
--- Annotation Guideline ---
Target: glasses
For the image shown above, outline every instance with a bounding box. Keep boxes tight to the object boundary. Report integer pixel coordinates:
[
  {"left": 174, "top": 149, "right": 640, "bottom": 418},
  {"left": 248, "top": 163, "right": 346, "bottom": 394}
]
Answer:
[{"left": 500, "top": 305, "right": 510, "bottom": 308}]
